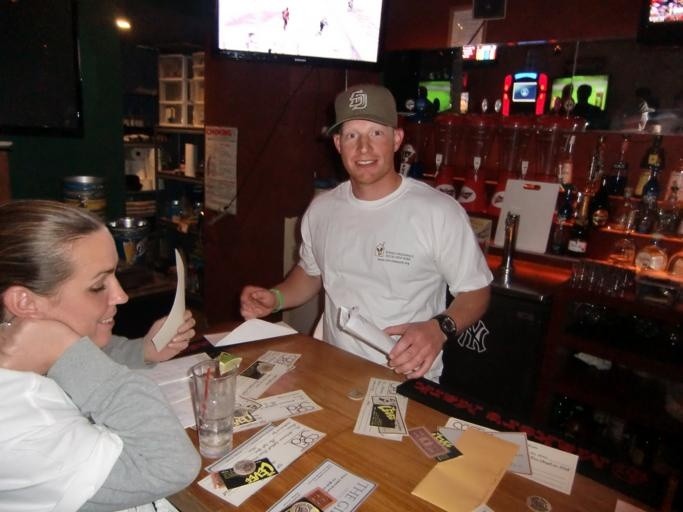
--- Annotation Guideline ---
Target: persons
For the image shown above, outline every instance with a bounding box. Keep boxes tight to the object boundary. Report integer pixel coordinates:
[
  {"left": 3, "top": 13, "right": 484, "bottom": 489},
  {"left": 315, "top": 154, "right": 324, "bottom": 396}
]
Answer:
[
  {"left": 555, "top": 85, "right": 575, "bottom": 113},
  {"left": 0, "top": 199, "right": 203, "bottom": 512},
  {"left": 569, "top": 86, "right": 602, "bottom": 117},
  {"left": 240, "top": 83, "right": 494, "bottom": 385}
]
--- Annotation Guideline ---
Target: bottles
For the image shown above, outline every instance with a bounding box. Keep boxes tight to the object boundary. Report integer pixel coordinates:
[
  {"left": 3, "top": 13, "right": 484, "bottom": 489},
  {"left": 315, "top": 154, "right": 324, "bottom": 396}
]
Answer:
[{"left": 551, "top": 134, "right": 683, "bottom": 280}]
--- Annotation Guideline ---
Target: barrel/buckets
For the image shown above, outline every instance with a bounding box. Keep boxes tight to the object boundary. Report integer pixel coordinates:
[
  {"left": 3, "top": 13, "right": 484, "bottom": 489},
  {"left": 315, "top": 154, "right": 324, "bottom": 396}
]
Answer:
[
  {"left": 105, "top": 217, "right": 148, "bottom": 275},
  {"left": 62, "top": 175, "right": 106, "bottom": 218}
]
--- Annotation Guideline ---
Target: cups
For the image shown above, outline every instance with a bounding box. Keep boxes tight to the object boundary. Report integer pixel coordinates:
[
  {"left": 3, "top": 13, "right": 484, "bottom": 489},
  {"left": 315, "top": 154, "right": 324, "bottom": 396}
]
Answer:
[{"left": 191, "top": 359, "right": 237, "bottom": 459}]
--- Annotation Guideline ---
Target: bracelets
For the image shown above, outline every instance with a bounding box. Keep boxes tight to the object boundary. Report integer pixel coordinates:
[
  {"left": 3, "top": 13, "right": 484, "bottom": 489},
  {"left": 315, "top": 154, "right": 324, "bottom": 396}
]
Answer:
[{"left": 269, "top": 288, "right": 285, "bottom": 315}]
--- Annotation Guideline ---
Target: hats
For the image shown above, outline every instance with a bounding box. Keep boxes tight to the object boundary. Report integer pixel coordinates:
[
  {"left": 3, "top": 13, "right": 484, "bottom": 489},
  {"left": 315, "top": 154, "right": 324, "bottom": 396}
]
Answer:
[{"left": 328, "top": 84, "right": 398, "bottom": 136}]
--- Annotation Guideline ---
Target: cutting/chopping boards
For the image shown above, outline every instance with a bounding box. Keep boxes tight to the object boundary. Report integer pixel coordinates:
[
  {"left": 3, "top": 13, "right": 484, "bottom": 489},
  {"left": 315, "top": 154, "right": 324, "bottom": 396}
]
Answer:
[{"left": 494, "top": 179, "right": 561, "bottom": 254}]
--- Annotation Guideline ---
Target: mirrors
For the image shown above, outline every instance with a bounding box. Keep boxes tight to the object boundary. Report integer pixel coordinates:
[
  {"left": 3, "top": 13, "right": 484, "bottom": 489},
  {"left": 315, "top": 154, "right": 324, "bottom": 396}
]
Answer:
[{"left": 0, "top": 0, "right": 85, "bottom": 138}]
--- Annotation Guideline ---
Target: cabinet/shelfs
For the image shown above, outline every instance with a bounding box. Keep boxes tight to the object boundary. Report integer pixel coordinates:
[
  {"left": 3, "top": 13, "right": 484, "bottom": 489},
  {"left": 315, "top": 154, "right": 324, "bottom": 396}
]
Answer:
[
  {"left": 395, "top": 121, "right": 683, "bottom": 480},
  {"left": 153, "top": 52, "right": 206, "bottom": 264}
]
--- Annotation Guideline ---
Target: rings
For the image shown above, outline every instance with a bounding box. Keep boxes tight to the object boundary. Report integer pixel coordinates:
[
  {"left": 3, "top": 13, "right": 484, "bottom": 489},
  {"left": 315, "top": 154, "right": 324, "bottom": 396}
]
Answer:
[{"left": 0, "top": 321, "right": 12, "bottom": 328}]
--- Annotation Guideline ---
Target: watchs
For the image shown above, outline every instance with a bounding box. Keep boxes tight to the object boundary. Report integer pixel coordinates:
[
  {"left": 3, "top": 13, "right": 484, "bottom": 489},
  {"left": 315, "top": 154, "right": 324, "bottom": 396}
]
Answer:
[{"left": 432, "top": 314, "right": 457, "bottom": 344}]
